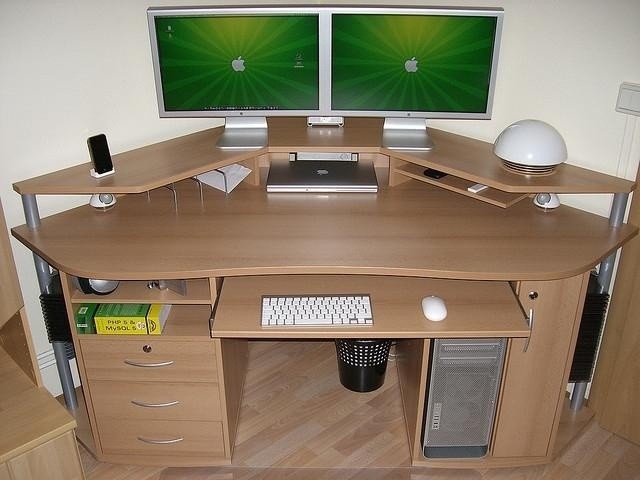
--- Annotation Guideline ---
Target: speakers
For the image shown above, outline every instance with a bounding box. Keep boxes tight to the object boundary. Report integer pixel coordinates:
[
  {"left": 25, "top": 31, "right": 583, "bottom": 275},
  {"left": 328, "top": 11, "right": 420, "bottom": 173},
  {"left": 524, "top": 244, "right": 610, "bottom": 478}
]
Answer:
[
  {"left": 90, "top": 193, "right": 116, "bottom": 207},
  {"left": 533, "top": 193, "right": 560, "bottom": 209}
]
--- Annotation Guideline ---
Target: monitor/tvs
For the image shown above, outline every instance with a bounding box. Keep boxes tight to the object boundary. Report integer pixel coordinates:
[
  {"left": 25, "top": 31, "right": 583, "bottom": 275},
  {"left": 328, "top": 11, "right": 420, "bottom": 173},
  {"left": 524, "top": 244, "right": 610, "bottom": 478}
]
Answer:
[
  {"left": 324, "top": 6, "right": 505, "bottom": 150},
  {"left": 146, "top": 7, "right": 324, "bottom": 151}
]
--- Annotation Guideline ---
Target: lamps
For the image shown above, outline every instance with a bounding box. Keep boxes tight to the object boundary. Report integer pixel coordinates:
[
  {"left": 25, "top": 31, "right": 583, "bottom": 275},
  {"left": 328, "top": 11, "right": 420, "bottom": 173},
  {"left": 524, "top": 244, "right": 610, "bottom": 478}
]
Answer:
[{"left": 491, "top": 118, "right": 569, "bottom": 180}]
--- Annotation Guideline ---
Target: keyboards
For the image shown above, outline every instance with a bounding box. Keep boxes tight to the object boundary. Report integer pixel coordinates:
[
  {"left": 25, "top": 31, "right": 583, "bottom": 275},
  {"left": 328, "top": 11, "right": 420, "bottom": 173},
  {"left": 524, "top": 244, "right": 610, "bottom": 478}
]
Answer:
[{"left": 261, "top": 294, "right": 373, "bottom": 326}]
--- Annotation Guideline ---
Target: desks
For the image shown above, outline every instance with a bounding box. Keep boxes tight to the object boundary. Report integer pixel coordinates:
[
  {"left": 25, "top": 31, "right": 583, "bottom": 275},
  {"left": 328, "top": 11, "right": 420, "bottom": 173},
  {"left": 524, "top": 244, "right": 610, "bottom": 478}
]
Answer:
[
  {"left": 9, "top": 116, "right": 639, "bottom": 471},
  {"left": 0, "top": 205, "right": 88, "bottom": 478}
]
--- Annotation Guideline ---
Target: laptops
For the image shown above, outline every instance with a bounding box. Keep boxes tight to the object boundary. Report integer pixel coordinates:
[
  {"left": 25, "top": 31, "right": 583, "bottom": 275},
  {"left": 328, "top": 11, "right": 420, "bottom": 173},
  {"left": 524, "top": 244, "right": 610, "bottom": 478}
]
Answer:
[{"left": 266, "top": 159, "right": 379, "bottom": 193}]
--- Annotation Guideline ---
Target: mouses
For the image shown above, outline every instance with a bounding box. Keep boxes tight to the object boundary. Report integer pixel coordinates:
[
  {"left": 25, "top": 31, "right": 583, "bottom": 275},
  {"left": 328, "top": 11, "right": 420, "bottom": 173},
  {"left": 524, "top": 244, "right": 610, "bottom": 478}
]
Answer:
[{"left": 421, "top": 295, "right": 447, "bottom": 321}]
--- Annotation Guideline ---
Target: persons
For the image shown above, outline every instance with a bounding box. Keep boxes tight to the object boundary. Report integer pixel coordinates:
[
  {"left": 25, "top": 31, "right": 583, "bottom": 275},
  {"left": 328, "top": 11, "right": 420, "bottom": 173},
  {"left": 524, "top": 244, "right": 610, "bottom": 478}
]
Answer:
[{"left": 74, "top": 301, "right": 174, "bottom": 335}]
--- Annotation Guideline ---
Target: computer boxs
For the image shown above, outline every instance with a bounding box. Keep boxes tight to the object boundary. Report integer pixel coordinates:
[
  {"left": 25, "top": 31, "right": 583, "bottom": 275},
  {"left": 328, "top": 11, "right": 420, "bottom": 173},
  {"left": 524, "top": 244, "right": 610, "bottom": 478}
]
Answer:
[{"left": 422, "top": 337, "right": 507, "bottom": 460}]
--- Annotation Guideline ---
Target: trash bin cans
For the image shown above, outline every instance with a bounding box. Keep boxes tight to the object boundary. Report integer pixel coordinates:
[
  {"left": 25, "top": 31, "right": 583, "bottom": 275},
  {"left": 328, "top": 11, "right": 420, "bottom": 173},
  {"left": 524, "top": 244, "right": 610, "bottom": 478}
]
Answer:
[{"left": 334, "top": 338, "right": 392, "bottom": 393}]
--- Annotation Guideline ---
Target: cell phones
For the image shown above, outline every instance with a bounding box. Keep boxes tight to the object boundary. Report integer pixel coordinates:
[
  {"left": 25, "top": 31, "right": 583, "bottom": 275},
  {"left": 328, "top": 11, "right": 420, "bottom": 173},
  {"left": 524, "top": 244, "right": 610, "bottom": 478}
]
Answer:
[{"left": 87, "top": 133, "right": 112, "bottom": 174}]
